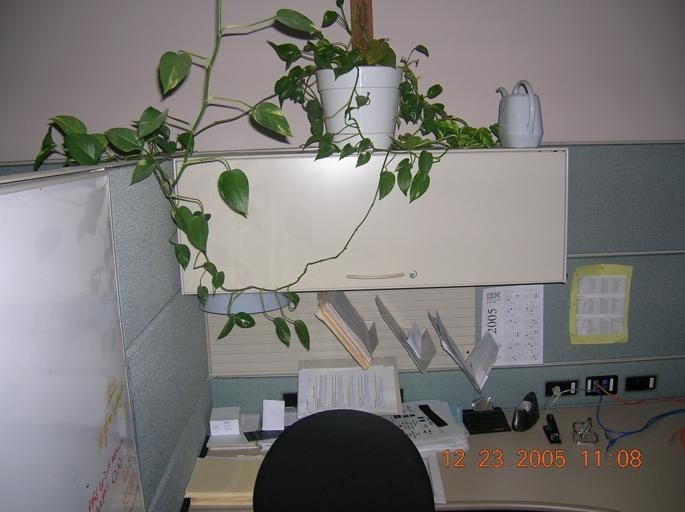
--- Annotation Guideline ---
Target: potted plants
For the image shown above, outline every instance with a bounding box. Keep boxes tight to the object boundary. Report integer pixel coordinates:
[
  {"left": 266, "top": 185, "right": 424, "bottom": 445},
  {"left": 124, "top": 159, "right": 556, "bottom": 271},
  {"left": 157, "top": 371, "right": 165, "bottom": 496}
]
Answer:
[{"left": 30, "top": 0, "right": 500, "bottom": 351}]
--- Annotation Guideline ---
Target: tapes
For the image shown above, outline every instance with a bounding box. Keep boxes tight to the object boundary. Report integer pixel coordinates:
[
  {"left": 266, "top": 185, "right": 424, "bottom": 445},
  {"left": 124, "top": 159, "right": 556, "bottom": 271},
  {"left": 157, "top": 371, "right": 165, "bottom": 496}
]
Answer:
[{"left": 520, "top": 400, "right": 532, "bottom": 412}]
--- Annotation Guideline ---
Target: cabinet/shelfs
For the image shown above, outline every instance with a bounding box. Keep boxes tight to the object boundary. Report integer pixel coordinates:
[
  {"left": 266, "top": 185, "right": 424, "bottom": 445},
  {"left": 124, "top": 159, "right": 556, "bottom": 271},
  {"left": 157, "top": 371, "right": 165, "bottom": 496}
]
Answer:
[{"left": 173, "top": 143, "right": 570, "bottom": 298}]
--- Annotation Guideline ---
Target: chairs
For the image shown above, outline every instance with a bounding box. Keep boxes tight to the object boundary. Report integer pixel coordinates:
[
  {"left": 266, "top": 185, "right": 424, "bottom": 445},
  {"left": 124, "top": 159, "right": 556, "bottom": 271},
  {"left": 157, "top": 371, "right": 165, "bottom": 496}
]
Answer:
[{"left": 253, "top": 410, "right": 436, "bottom": 512}]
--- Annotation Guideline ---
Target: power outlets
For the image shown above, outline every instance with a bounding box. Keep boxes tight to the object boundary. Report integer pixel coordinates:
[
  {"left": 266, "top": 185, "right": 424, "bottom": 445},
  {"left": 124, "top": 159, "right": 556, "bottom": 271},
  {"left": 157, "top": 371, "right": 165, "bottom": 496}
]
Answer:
[
  {"left": 586, "top": 376, "right": 618, "bottom": 395},
  {"left": 544, "top": 381, "right": 578, "bottom": 397}
]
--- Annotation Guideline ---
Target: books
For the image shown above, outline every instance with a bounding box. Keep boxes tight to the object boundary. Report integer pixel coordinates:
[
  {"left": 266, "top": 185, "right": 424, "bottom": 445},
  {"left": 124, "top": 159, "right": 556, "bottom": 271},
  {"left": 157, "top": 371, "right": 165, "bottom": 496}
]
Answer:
[
  {"left": 314, "top": 291, "right": 375, "bottom": 370},
  {"left": 205, "top": 413, "right": 260, "bottom": 450}
]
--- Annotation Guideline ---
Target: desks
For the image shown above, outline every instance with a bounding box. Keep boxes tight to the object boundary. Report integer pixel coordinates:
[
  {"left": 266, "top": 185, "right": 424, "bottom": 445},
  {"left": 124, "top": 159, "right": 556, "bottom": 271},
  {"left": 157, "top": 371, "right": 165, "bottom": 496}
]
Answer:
[{"left": 186, "top": 398, "right": 685, "bottom": 512}]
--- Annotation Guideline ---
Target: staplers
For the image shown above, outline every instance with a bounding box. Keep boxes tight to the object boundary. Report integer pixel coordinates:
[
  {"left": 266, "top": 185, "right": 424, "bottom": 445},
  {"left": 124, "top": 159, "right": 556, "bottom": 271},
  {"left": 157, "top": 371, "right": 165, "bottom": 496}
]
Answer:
[{"left": 543, "top": 414, "right": 562, "bottom": 444}]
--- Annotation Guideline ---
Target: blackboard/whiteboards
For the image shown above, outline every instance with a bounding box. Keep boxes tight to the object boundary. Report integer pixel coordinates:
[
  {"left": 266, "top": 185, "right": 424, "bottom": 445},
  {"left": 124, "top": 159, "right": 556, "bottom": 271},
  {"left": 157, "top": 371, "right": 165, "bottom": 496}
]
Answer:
[{"left": 0, "top": 169, "right": 147, "bottom": 512}]
